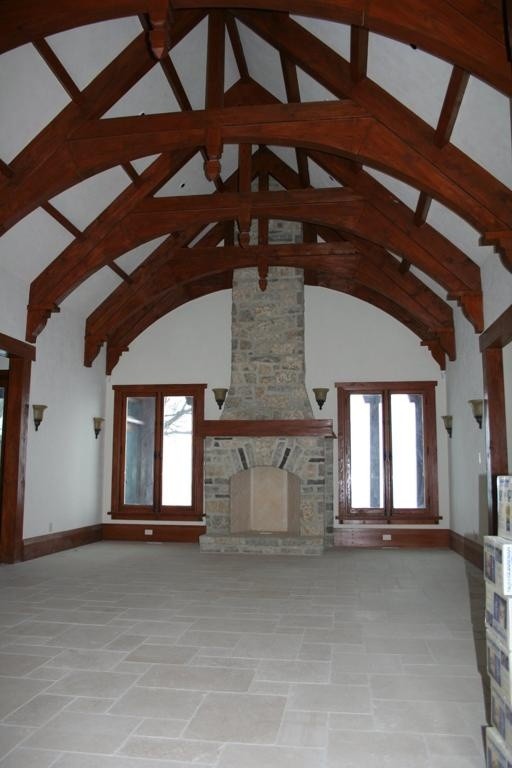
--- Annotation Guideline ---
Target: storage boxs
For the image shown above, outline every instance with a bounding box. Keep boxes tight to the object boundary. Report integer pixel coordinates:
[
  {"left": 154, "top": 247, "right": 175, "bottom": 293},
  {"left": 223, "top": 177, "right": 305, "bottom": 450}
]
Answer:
[{"left": 482, "top": 475, "right": 512, "bottom": 768}]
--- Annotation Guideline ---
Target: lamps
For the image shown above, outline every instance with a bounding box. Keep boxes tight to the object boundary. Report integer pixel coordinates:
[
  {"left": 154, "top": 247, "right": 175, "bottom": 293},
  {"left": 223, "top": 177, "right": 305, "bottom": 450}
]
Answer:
[
  {"left": 442, "top": 416, "right": 452, "bottom": 438},
  {"left": 33, "top": 405, "right": 48, "bottom": 431},
  {"left": 212, "top": 388, "right": 228, "bottom": 409},
  {"left": 94, "top": 418, "right": 105, "bottom": 438},
  {"left": 468, "top": 399, "right": 482, "bottom": 429},
  {"left": 312, "top": 388, "right": 330, "bottom": 410}
]
